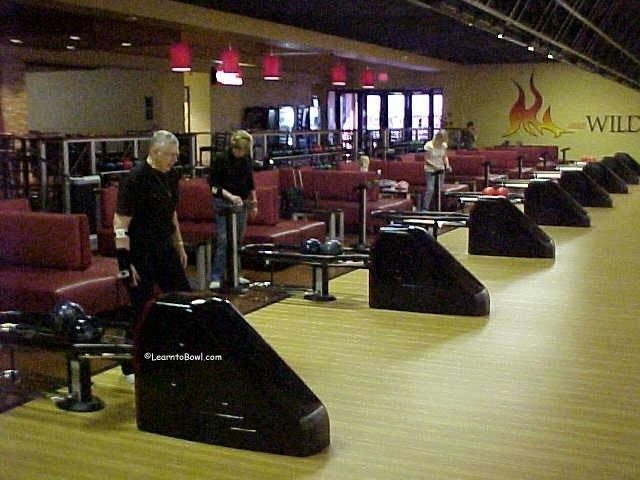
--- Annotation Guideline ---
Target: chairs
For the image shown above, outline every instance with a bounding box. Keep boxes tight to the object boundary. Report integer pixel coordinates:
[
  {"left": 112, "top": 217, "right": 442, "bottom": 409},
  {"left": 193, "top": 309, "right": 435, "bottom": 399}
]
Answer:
[
  {"left": 199, "top": 131, "right": 228, "bottom": 165},
  {"left": 296, "top": 144, "right": 562, "bottom": 225}
]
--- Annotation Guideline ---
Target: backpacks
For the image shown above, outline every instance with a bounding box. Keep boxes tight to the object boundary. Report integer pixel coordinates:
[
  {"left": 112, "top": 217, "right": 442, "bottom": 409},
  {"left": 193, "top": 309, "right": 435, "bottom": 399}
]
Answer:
[{"left": 280, "top": 186, "right": 302, "bottom": 219}]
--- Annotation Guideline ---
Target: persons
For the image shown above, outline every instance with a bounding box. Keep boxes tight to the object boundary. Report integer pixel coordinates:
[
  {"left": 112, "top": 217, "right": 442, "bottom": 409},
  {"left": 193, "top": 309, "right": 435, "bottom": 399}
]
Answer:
[
  {"left": 422, "top": 129, "right": 453, "bottom": 210},
  {"left": 460, "top": 120, "right": 479, "bottom": 150},
  {"left": 110, "top": 129, "right": 193, "bottom": 382},
  {"left": 209, "top": 130, "right": 258, "bottom": 291}
]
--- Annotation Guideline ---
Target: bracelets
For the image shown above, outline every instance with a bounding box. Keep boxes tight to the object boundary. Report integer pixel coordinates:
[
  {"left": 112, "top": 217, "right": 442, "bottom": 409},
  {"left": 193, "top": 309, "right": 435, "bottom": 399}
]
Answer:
[
  {"left": 175, "top": 240, "right": 183, "bottom": 247},
  {"left": 251, "top": 200, "right": 257, "bottom": 208}
]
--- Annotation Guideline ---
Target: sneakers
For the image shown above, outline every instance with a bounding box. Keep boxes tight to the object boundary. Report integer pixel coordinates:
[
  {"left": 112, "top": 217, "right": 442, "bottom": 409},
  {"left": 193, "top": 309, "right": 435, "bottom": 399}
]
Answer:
[{"left": 208, "top": 276, "right": 251, "bottom": 289}]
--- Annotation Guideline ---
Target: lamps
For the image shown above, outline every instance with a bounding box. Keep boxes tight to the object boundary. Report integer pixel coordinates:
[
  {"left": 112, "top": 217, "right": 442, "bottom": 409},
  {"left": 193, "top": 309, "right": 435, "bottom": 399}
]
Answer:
[
  {"left": 332, "top": 56, "right": 376, "bottom": 90},
  {"left": 168, "top": 24, "right": 283, "bottom": 88}
]
